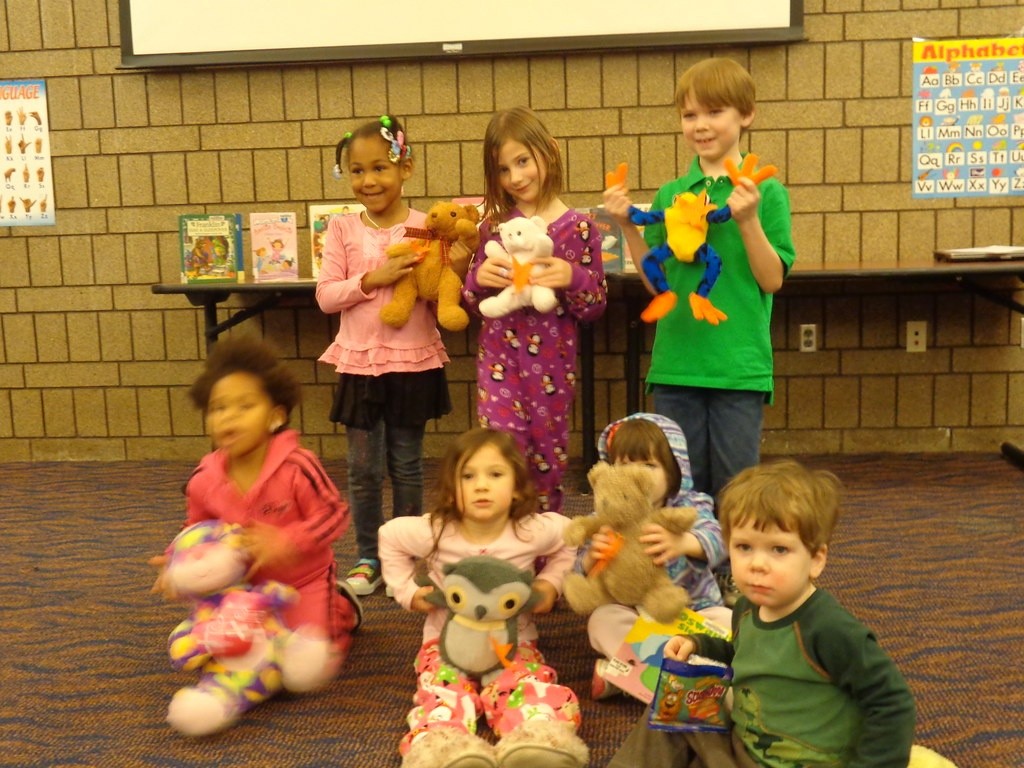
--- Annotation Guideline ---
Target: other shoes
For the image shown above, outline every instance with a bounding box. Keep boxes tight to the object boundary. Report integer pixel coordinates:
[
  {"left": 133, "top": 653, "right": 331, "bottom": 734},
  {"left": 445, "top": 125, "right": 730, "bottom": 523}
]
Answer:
[
  {"left": 398, "top": 726, "right": 498, "bottom": 767},
  {"left": 495, "top": 720, "right": 590, "bottom": 768}
]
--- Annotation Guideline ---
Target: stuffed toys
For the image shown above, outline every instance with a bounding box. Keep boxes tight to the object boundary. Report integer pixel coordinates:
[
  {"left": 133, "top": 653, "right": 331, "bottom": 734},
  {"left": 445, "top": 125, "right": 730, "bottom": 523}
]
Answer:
[
  {"left": 561, "top": 461, "right": 697, "bottom": 621},
  {"left": 479, "top": 214, "right": 557, "bottom": 317},
  {"left": 411, "top": 556, "right": 536, "bottom": 681},
  {"left": 384, "top": 201, "right": 476, "bottom": 331},
  {"left": 151, "top": 518, "right": 340, "bottom": 738},
  {"left": 605, "top": 150, "right": 778, "bottom": 323}
]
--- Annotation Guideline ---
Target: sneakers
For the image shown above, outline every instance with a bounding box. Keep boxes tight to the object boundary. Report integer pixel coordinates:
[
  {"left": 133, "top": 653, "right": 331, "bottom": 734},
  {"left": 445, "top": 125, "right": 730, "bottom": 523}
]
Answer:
[
  {"left": 343, "top": 553, "right": 384, "bottom": 596},
  {"left": 591, "top": 658, "right": 624, "bottom": 701},
  {"left": 334, "top": 579, "right": 363, "bottom": 637}
]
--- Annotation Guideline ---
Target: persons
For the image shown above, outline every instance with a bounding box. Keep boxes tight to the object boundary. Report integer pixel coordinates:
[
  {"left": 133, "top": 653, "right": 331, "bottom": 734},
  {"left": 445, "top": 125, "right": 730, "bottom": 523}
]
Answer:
[
  {"left": 375, "top": 428, "right": 590, "bottom": 767},
  {"left": 462, "top": 106, "right": 604, "bottom": 576},
  {"left": 589, "top": 410, "right": 731, "bottom": 706},
  {"left": 146, "top": 338, "right": 362, "bottom": 692},
  {"left": 599, "top": 56, "right": 795, "bottom": 522},
  {"left": 603, "top": 461, "right": 916, "bottom": 768},
  {"left": 315, "top": 117, "right": 473, "bottom": 592}
]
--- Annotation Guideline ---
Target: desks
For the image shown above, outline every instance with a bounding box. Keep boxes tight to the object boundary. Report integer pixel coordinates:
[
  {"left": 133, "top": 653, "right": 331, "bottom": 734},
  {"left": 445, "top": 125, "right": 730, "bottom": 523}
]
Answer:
[{"left": 150, "top": 258, "right": 1024, "bottom": 493}]
